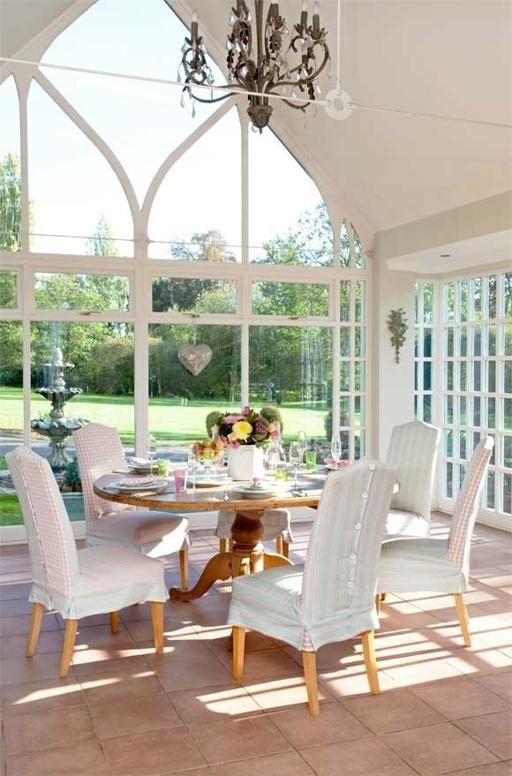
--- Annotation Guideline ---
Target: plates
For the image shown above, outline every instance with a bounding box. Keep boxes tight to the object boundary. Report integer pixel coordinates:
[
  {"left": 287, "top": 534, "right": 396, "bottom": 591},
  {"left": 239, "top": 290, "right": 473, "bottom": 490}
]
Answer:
[
  {"left": 128, "top": 464, "right": 160, "bottom": 473},
  {"left": 324, "top": 463, "right": 342, "bottom": 472},
  {"left": 106, "top": 479, "right": 167, "bottom": 495},
  {"left": 232, "top": 480, "right": 278, "bottom": 498}
]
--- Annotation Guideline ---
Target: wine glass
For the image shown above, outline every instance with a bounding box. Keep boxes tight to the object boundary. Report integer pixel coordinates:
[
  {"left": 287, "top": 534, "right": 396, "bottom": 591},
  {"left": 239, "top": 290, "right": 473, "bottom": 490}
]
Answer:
[{"left": 145, "top": 430, "right": 343, "bottom": 501}]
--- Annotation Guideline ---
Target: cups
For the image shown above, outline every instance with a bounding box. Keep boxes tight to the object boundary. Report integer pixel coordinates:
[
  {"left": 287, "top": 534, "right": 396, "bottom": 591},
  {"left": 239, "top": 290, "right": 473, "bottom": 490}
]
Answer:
[
  {"left": 275, "top": 451, "right": 318, "bottom": 483},
  {"left": 156, "top": 456, "right": 188, "bottom": 493}
]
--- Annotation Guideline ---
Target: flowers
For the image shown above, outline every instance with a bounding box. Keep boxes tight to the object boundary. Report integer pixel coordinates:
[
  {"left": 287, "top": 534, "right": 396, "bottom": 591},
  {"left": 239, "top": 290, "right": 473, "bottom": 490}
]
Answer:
[{"left": 194, "top": 405, "right": 283, "bottom": 461}]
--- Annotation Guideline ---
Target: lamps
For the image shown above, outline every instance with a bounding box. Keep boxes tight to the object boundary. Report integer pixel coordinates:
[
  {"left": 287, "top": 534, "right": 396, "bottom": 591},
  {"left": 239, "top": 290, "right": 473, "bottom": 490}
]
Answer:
[{"left": 177, "top": 2, "right": 336, "bottom": 133}]
